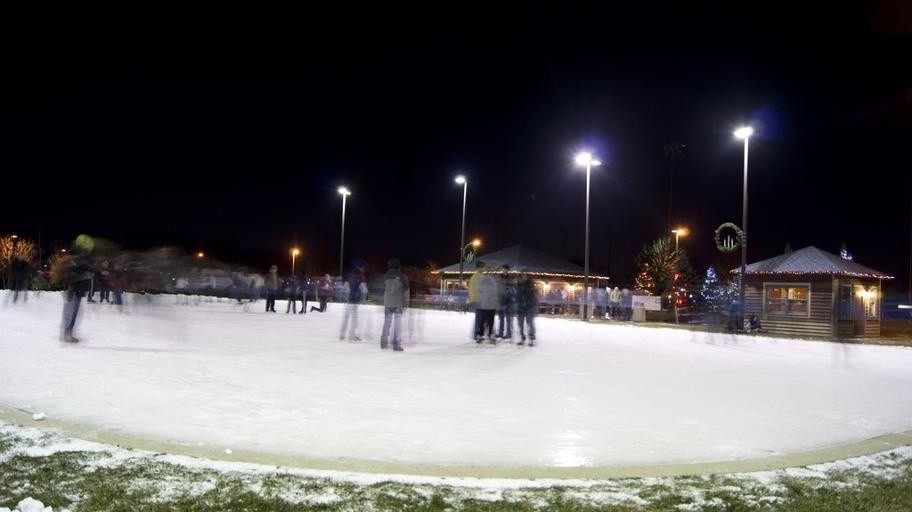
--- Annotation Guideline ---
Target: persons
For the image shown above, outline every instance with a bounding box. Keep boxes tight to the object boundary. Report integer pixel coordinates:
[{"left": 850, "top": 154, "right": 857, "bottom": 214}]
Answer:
[
  {"left": 744, "top": 315, "right": 768, "bottom": 333},
  {"left": 728, "top": 298, "right": 741, "bottom": 332}
]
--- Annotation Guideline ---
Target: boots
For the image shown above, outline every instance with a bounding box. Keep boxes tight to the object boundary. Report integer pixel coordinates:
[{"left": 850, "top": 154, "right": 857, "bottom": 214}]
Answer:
[
  {"left": 380, "top": 336, "right": 406, "bottom": 352},
  {"left": 336, "top": 331, "right": 368, "bottom": 344},
  {"left": 474, "top": 328, "right": 537, "bottom": 346}
]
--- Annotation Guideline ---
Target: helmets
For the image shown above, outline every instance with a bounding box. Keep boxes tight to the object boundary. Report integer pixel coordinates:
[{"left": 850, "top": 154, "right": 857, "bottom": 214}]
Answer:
[{"left": 75, "top": 233, "right": 95, "bottom": 251}]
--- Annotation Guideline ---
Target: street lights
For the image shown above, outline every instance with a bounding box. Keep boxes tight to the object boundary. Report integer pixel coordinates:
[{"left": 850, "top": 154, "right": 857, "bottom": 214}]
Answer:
[
  {"left": 290, "top": 247, "right": 300, "bottom": 284},
  {"left": 337, "top": 184, "right": 352, "bottom": 285},
  {"left": 453, "top": 174, "right": 482, "bottom": 291},
  {"left": 733, "top": 126, "right": 755, "bottom": 333},
  {"left": 573, "top": 150, "right": 603, "bottom": 323},
  {"left": 670, "top": 228, "right": 688, "bottom": 252}
]
